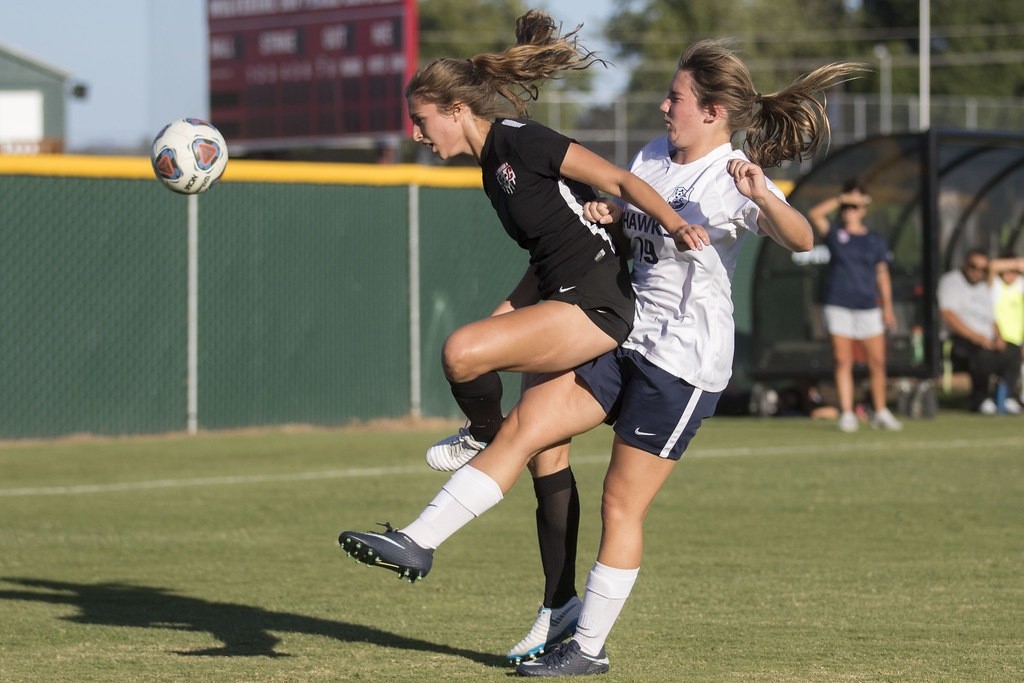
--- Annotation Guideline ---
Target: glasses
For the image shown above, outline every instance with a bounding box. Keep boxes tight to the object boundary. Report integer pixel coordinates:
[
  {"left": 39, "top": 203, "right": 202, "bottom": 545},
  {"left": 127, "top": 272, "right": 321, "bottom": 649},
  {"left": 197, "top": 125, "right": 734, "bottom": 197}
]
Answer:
[
  {"left": 840, "top": 203, "right": 860, "bottom": 211},
  {"left": 965, "top": 263, "right": 988, "bottom": 274}
]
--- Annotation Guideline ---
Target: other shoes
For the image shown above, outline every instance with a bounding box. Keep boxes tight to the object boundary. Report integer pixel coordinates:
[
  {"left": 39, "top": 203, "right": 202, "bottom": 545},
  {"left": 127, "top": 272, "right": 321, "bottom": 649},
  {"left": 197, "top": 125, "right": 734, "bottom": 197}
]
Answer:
[
  {"left": 977, "top": 397, "right": 998, "bottom": 416},
  {"left": 838, "top": 410, "right": 858, "bottom": 433},
  {"left": 1003, "top": 397, "right": 1021, "bottom": 415},
  {"left": 870, "top": 408, "right": 903, "bottom": 432}
]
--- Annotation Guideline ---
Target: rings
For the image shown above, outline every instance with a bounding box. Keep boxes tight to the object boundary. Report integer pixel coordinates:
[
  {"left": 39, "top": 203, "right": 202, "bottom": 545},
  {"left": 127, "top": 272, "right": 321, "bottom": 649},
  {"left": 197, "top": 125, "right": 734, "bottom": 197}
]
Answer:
[{"left": 687, "top": 230, "right": 693, "bottom": 233}]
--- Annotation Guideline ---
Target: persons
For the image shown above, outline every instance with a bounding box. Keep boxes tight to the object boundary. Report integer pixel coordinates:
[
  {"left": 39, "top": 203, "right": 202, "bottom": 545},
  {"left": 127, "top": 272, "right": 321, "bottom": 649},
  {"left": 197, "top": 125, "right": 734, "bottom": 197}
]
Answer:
[
  {"left": 339, "top": 40, "right": 870, "bottom": 675},
  {"left": 406, "top": 8, "right": 711, "bottom": 660},
  {"left": 804, "top": 177, "right": 903, "bottom": 430},
  {"left": 936, "top": 246, "right": 1024, "bottom": 416}
]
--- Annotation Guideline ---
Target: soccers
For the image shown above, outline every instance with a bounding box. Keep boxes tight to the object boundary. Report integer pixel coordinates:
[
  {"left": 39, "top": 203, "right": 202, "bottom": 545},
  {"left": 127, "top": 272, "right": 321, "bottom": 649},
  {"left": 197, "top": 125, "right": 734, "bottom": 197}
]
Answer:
[{"left": 149, "top": 116, "right": 230, "bottom": 195}]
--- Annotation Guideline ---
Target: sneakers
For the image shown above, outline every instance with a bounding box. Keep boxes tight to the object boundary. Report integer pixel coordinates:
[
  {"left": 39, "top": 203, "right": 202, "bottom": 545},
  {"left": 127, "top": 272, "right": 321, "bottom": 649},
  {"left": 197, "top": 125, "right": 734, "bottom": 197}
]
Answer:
[
  {"left": 425, "top": 419, "right": 488, "bottom": 471},
  {"left": 516, "top": 639, "right": 610, "bottom": 677},
  {"left": 338, "top": 522, "right": 434, "bottom": 584},
  {"left": 504, "top": 596, "right": 583, "bottom": 663}
]
